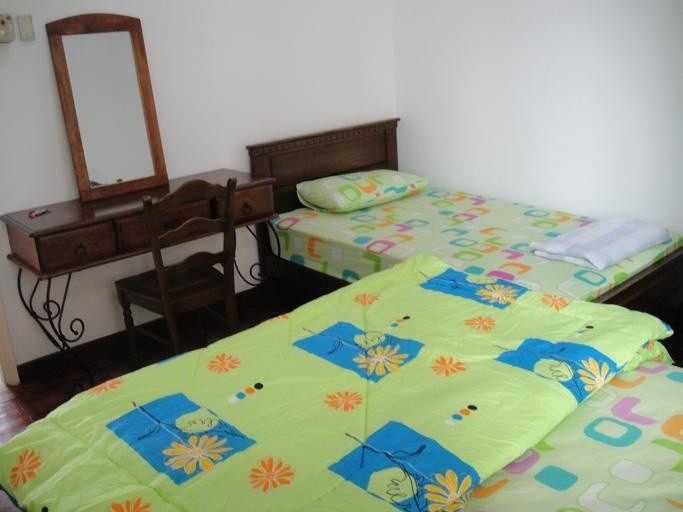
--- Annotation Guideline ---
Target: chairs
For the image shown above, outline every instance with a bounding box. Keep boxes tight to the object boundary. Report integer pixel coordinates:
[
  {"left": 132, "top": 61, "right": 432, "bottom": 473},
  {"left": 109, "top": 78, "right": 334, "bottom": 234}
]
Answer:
[{"left": 112, "top": 177, "right": 237, "bottom": 361}]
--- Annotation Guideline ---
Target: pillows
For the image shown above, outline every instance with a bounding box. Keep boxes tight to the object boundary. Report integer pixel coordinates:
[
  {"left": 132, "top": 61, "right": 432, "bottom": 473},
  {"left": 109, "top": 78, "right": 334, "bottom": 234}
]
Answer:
[{"left": 295, "top": 167, "right": 429, "bottom": 214}]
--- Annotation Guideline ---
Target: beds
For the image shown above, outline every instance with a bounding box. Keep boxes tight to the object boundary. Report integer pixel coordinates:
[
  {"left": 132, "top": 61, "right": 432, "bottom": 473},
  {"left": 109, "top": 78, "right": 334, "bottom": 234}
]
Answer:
[
  {"left": 1, "top": 258, "right": 683, "bottom": 512},
  {"left": 247, "top": 118, "right": 682, "bottom": 302}
]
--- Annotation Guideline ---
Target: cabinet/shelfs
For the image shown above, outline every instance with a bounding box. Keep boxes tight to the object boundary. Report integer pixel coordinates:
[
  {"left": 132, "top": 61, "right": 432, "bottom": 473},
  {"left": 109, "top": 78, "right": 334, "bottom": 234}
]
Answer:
[{"left": 0, "top": 170, "right": 279, "bottom": 392}]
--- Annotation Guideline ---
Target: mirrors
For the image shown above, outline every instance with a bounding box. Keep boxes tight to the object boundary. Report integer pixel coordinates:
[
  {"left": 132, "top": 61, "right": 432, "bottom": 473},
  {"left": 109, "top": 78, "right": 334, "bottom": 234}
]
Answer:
[{"left": 43, "top": 15, "right": 168, "bottom": 201}]
